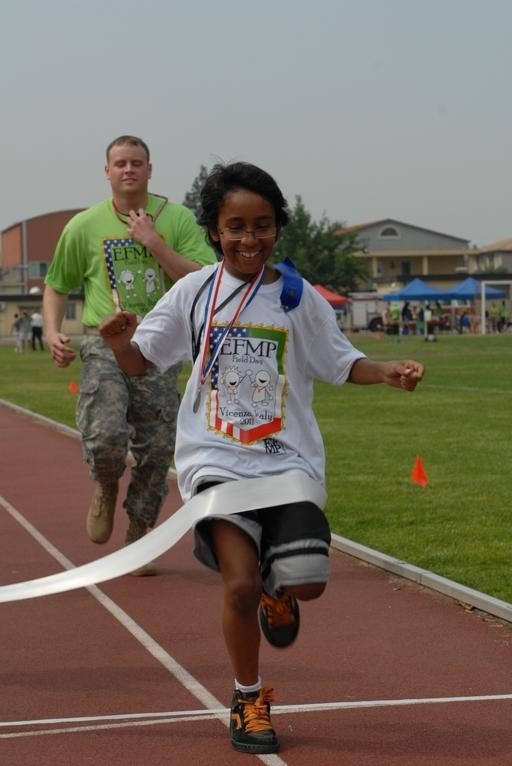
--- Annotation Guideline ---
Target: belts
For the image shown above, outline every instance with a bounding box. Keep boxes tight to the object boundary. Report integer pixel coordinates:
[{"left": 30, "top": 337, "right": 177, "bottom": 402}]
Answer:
[{"left": 82, "top": 326, "right": 103, "bottom": 336}]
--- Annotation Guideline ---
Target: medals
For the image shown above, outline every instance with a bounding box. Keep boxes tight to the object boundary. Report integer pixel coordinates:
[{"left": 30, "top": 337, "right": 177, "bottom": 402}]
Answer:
[{"left": 192, "top": 389, "right": 204, "bottom": 412}]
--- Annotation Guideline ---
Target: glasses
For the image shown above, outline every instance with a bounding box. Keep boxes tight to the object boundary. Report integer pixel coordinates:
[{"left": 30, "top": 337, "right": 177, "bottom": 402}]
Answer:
[{"left": 215, "top": 223, "right": 276, "bottom": 241}]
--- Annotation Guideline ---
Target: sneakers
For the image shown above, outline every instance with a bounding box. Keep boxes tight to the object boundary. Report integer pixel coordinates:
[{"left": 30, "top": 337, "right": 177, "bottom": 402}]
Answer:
[
  {"left": 258, "top": 588, "right": 301, "bottom": 649},
  {"left": 227, "top": 688, "right": 280, "bottom": 754}
]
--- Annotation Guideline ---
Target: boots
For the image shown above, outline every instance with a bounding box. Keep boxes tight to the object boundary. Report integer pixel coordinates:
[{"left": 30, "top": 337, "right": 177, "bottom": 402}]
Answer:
[
  {"left": 124, "top": 521, "right": 158, "bottom": 578},
  {"left": 86, "top": 480, "right": 120, "bottom": 545}
]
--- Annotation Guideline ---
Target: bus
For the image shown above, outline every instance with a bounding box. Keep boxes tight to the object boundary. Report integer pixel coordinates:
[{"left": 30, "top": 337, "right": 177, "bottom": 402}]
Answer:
[{"left": 349, "top": 292, "right": 471, "bottom": 332}]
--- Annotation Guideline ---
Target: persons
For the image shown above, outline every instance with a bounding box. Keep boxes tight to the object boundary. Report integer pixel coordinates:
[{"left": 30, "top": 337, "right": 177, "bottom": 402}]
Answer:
[
  {"left": 16, "top": 310, "right": 32, "bottom": 353},
  {"left": 31, "top": 310, "right": 45, "bottom": 351},
  {"left": 10, "top": 313, "right": 25, "bottom": 352},
  {"left": 97, "top": 161, "right": 427, "bottom": 753},
  {"left": 43, "top": 133, "right": 217, "bottom": 575},
  {"left": 381, "top": 300, "right": 511, "bottom": 341}
]
